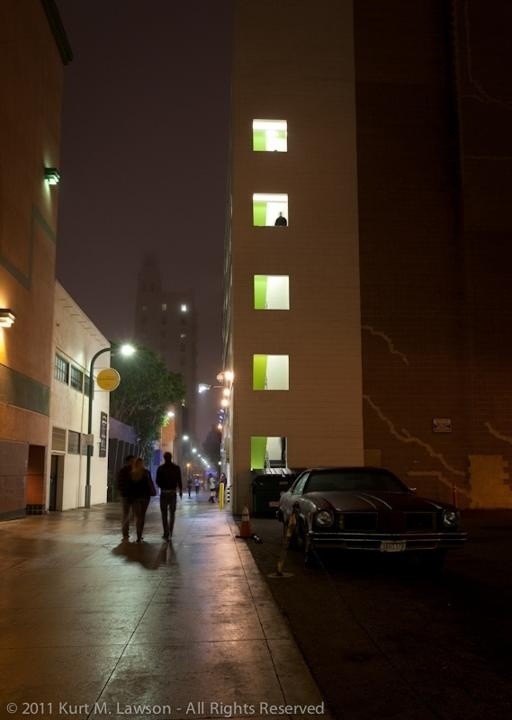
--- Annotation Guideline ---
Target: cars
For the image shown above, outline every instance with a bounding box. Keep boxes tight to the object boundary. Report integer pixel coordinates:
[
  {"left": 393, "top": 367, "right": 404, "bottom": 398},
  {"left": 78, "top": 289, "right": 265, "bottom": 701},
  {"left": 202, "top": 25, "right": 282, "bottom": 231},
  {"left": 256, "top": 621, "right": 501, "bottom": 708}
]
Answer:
[{"left": 279, "top": 465, "right": 470, "bottom": 566}]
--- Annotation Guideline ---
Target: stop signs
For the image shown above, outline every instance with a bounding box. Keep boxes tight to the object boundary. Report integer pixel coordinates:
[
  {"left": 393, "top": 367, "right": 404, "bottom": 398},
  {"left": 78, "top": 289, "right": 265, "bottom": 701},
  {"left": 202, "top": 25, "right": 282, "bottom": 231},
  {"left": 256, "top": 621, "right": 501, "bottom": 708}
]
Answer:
[{"left": 96, "top": 368, "right": 120, "bottom": 391}]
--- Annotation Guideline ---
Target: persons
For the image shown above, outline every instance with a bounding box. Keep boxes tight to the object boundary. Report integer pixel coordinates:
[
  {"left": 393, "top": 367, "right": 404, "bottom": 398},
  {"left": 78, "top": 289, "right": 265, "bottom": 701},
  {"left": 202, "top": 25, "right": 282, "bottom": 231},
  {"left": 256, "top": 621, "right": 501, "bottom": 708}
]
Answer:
[
  {"left": 195, "top": 476, "right": 200, "bottom": 496},
  {"left": 274, "top": 212, "right": 287, "bottom": 226},
  {"left": 187, "top": 476, "right": 192, "bottom": 496},
  {"left": 220, "top": 473, "right": 227, "bottom": 487},
  {"left": 130, "top": 457, "right": 157, "bottom": 543},
  {"left": 115, "top": 455, "right": 136, "bottom": 538},
  {"left": 207, "top": 473, "right": 216, "bottom": 503},
  {"left": 156, "top": 452, "right": 183, "bottom": 543}
]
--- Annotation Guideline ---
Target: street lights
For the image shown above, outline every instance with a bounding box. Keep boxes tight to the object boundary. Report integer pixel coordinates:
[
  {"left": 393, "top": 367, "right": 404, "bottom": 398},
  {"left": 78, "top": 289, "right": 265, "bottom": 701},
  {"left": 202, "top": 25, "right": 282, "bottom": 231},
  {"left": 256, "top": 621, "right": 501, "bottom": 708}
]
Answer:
[
  {"left": 83, "top": 342, "right": 148, "bottom": 511},
  {"left": 159, "top": 410, "right": 211, "bottom": 490}
]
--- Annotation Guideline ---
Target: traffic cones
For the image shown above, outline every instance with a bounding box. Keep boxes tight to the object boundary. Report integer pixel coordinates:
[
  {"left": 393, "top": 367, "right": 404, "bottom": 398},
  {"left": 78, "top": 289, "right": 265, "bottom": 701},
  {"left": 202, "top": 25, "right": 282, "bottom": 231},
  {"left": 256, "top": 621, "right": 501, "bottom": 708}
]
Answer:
[{"left": 240, "top": 504, "right": 251, "bottom": 540}]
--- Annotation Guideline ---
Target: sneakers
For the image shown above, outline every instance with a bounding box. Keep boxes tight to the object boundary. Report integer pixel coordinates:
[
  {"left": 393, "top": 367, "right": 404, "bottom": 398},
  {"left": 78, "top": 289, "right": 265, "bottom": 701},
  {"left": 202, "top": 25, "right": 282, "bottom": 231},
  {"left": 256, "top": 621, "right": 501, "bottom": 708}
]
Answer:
[{"left": 121, "top": 532, "right": 173, "bottom": 544}]
